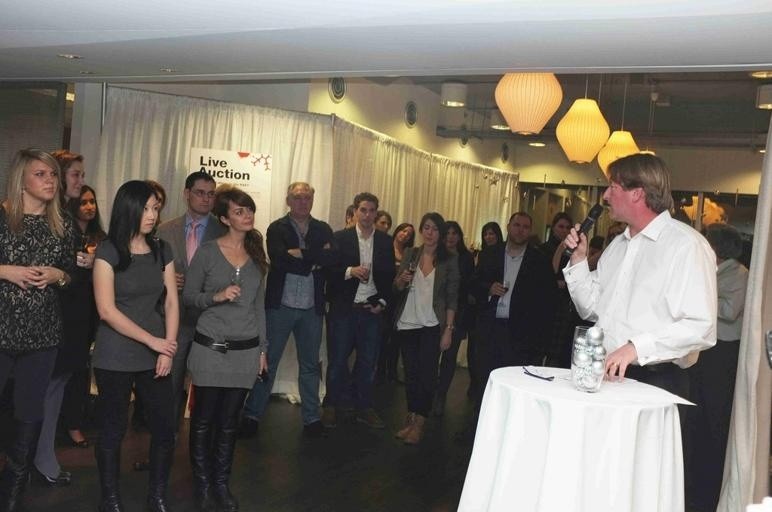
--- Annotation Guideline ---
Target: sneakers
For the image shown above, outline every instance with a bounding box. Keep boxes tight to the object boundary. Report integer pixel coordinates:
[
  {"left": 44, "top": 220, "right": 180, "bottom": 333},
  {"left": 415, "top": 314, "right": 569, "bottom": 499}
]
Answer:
[
  {"left": 320, "top": 405, "right": 338, "bottom": 429},
  {"left": 354, "top": 408, "right": 387, "bottom": 430}
]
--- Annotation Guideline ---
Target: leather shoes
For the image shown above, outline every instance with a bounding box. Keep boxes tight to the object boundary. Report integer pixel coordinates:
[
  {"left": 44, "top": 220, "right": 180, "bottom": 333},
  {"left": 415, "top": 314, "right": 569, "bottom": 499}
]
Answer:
[
  {"left": 301, "top": 422, "right": 336, "bottom": 439},
  {"left": 234, "top": 421, "right": 258, "bottom": 439}
]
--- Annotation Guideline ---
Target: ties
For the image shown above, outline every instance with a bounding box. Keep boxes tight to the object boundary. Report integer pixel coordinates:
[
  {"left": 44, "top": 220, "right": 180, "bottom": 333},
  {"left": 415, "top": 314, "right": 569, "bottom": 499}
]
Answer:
[{"left": 186, "top": 221, "right": 199, "bottom": 266}]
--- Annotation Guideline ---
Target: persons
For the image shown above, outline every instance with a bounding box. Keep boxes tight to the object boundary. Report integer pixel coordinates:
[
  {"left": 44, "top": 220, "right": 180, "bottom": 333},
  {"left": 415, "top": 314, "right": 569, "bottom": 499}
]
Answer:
[
  {"left": 1, "top": 150, "right": 271, "bottom": 512},
  {"left": 562, "top": 152, "right": 754, "bottom": 511},
  {"left": 240, "top": 184, "right": 628, "bottom": 446}
]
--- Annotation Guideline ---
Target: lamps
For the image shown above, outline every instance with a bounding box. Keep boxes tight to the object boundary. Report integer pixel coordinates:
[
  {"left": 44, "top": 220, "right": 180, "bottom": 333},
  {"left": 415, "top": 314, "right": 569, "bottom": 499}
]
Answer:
[
  {"left": 555, "top": 72, "right": 610, "bottom": 164},
  {"left": 490, "top": 107, "right": 510, "bottom": 131},
  {"left": 598, "top": 73, "right": 639, "bottom": 179},
  {"left": 441, "top": 83, "right": 468, "bottom": 107},
  {"left": 755, "top": 84, "right": 772, "bottom": 110},
  {"left": 493, "top": 74, "right": 563, "bottom": 135}
]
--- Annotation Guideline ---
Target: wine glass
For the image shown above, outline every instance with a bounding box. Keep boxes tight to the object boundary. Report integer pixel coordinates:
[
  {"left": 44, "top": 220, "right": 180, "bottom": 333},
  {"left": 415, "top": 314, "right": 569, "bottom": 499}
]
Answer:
[
  {"left": 498, "top": 280, "right": 510, "bottom": 306},
  {"left": 230, "top": 266, "right": 244, "bottom": 303},
  {"left": 404, "top": 262, "right": 414, "bottom": 289}
]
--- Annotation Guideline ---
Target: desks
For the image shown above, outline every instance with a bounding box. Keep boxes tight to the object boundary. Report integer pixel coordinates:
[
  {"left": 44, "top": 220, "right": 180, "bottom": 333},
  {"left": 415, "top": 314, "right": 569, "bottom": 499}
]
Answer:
[{"left": 453, "top": 367, "right": 685, "bottom": 512}]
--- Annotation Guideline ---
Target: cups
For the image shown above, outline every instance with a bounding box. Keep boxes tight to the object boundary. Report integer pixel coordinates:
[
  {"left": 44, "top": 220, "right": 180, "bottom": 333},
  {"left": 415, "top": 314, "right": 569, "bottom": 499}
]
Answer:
[
  {"left": 471, "top": 240, "right": 479, "bottom": 257},
  {"left": 174, "top": 258, "right": 186, "bottom": 274},
  {"left": 362, "top": 263, "right": 371, "bottom": 284},
  {"left": 570, "top": 325, "right": 611, "bottom": 393}
]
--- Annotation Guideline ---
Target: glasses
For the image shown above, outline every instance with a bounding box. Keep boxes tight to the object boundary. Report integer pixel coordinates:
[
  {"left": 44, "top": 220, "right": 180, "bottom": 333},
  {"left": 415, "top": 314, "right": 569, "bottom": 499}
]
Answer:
[{"left": 190, "top": 189, "right": 217, "bottom": 199}]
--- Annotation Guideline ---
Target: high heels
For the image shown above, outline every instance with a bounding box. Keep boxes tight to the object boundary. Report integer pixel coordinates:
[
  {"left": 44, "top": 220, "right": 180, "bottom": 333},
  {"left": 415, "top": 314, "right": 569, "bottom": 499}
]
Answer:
[
  {"left": 65, "top": 429, "right": 89, "bottom": 450},
  {"left": 25, "top": 457, "right": 72, "bottom": 488}
]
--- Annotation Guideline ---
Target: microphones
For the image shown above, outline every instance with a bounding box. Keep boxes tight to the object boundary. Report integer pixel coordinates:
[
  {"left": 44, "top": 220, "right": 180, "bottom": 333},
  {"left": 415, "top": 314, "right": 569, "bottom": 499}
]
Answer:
[{"left": 564, "top": 203, "right": 604, "bottom": 257}]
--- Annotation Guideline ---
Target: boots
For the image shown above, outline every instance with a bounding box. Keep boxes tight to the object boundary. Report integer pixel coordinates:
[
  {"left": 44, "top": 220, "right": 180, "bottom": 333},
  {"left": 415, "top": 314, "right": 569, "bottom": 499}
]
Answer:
[
  {"left": 434, "top": 392, "right": 447, "bottom": 417},
  {"left": 0, "top": 416, "right": 45, "bottom": 512},
  {"left": 93, "top": 416, "right": 124, "bottom": 512},
  {"left": 144, "top": 417, "right": 177, "bottom": 512},
  {"left": 396, "top": 411, "right": 418, "bottom": 440},
  {"left": 216, "top": 414, "right": 239, "bottom": 512},
  {"left": 189, "top": 415, "right": 217, "bottom": 512},
  {"left": 404, "top": 412, "right": 425, "bottom": 445}
]
